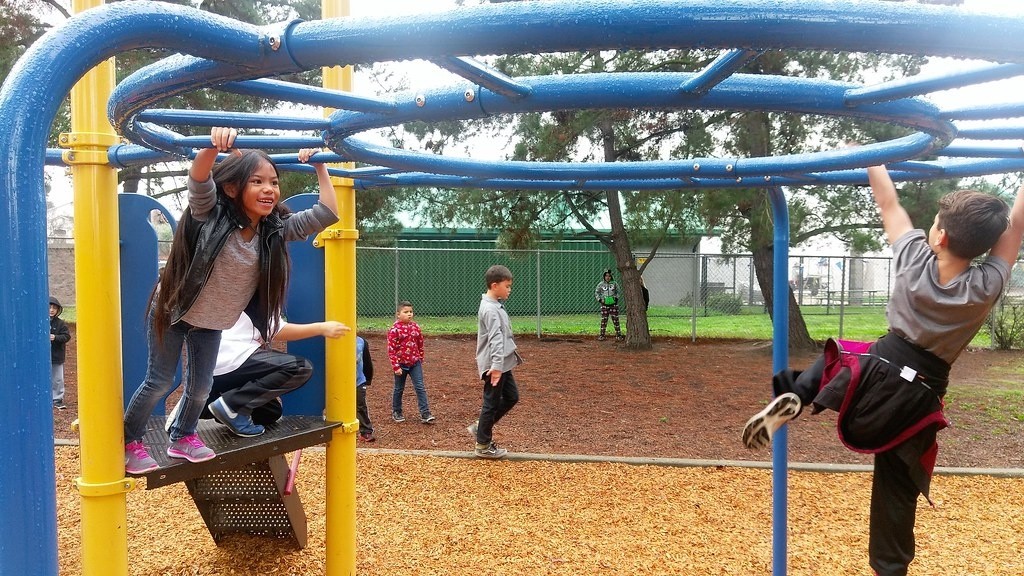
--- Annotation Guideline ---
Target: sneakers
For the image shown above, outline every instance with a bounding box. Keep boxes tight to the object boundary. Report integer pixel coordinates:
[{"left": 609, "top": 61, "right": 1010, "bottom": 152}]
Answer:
[
  {"left": 167, "top": 434, "right": 216, "bottom": 463},
  {"left": 124, "top": 440, "right": 161, "bottom": 474},
  {"left": 421, "top": 412, "right": 435, "bottom": 423},
  {"left": 54, "top": 402, "right": 67, "bottom": 409},
  {"left": 392, "top": 409, "right": 405, "bottom": 422},
  {"left": 467, "top": 424, "right": 478, "bottom": 440},
  {"left": 741, "top": 392, "right": 801, "bottom": 454},
  {"left": 359, "top": 433, "right": 375, "bottom": 441},
  {"left": 475, "top": 442, "right": 507, "bottom": 458},
  {"left": 208, "top": 396, "right": 265, "bottom": 437}
]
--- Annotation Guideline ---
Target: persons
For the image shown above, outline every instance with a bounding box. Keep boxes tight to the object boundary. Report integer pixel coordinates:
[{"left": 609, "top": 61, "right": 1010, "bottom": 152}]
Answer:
[
  {"left": 594, "top": 270, "right": 624, "bottom": 342},
  {"left": 740, "top": 166, "right": 1024, "bottom": 576},
  {"left": 641, "top": 277, "right": 650, "bottom": 311},
  {"left": 49, "top": 296, "right": 71, "bottom": 409},
  {"left": 465, "top": 265, "right": 523, "bottom": 459},
  {"left": 387, "top": 301, "right": 435, "bottom": 423},
  {"left": 164, "top": 303, "right": 352, "bottom": 438},
  {"left": 356, "top": 336, "right": 377, "bottom": 443},
  {"left": 124, "top": 125, "right": 341, "bottom": 475}
]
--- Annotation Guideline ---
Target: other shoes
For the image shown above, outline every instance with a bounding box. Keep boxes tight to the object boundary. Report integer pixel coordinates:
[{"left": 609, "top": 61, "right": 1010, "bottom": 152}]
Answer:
[
  {"left": 598, "top": 335, "right": 605, "bottom": 341},
  {"left": 616, "top": 336, "right": 623, "bottom": 341}
]
